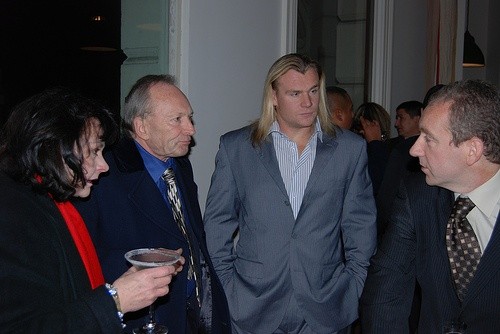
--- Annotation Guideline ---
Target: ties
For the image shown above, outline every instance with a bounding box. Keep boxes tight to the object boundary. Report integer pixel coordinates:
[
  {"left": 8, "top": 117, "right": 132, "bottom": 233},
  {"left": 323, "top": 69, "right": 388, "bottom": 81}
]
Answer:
[
  {"left": 446, "top": 196, "right": 482, "bottom": 302},
  {"left": 162, "top": 166, "right": 203, "bottom": 308}
]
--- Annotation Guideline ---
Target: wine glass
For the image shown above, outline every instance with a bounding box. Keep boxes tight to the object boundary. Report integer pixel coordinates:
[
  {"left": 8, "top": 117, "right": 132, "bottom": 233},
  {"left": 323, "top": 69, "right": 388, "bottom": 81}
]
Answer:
[
  {"left": 351, "top": 119, "right": 387, "bottom": 143},
  {"left": 124, "top": 248, "right": 180, "bottom": 334}
]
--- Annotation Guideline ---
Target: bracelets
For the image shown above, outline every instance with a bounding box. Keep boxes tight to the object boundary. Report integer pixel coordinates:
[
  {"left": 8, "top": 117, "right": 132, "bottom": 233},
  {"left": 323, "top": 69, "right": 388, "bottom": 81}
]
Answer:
[{"left": 105, "top": 283, "right": 123, "bottom": 320}]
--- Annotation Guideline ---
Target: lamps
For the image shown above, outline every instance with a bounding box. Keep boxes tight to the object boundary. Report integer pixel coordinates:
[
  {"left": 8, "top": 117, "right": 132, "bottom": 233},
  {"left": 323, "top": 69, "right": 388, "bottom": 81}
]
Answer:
[{"left": 462, "top": 0, "right": 486, "bottom": 67}]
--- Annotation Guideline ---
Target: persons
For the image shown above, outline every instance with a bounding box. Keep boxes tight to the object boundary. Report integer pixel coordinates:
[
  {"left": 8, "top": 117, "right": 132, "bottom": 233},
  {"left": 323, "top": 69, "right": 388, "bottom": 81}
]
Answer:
[
  {"left": 367, "top": 80, "right": 500, "bottom": 334},
  {"left": 0, "top": 88, "right": 186, "bottom": 334},
  {"left": 202, "top": 53, "right": 377, "bottom": 334},
  {"left": 324, "top": 84, "right": 446, "bottom": 247},
  {"left": 95, "top": 74, "right": 233, "bottom": 334}
]
